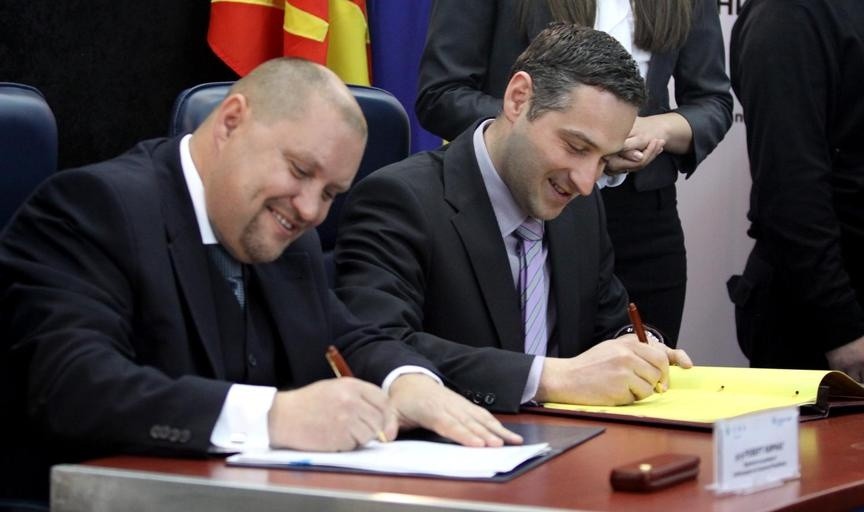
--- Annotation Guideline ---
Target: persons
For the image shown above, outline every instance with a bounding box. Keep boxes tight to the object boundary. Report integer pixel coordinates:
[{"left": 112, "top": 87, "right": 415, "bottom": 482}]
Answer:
[
  {"left": 0, "top": 51, "right": 533, "bottom": 512},
  {"left": 326, "top": 18, "right": 695, "bottom": 410},
  {"left": 412, "top": 1, "right": 732, "bottom": 372},
  {"left": 722, "top": 1, "right": 862, "bottom": 396}
]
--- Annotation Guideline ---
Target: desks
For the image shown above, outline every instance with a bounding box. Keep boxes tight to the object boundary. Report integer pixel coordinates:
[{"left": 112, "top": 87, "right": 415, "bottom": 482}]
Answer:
[{"left": 50, "top": 382, "right": 862, "bottom": 511}]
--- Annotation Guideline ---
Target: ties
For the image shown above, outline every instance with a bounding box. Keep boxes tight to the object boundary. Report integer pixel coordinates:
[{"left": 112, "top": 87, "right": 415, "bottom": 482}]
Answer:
[
  {"left": 513, "top": 215, "right": 547, "bottom": 356},
  {"left": 211, "top": 249, "right": 246, "bottom": 308}
]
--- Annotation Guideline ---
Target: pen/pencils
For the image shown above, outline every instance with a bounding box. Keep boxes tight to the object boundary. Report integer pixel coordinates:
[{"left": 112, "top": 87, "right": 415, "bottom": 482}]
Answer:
[
  {"left": 325, "top": 344, "right": 388, "bottom": 443},
  {"left": 627, "top": 302, "right": 664, "bottom": 395}
]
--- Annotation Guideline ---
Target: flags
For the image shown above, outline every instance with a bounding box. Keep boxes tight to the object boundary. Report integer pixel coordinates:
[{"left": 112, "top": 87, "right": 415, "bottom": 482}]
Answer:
[{"left": 209, "top": 2, "right": 376, "bottom": 93}]
